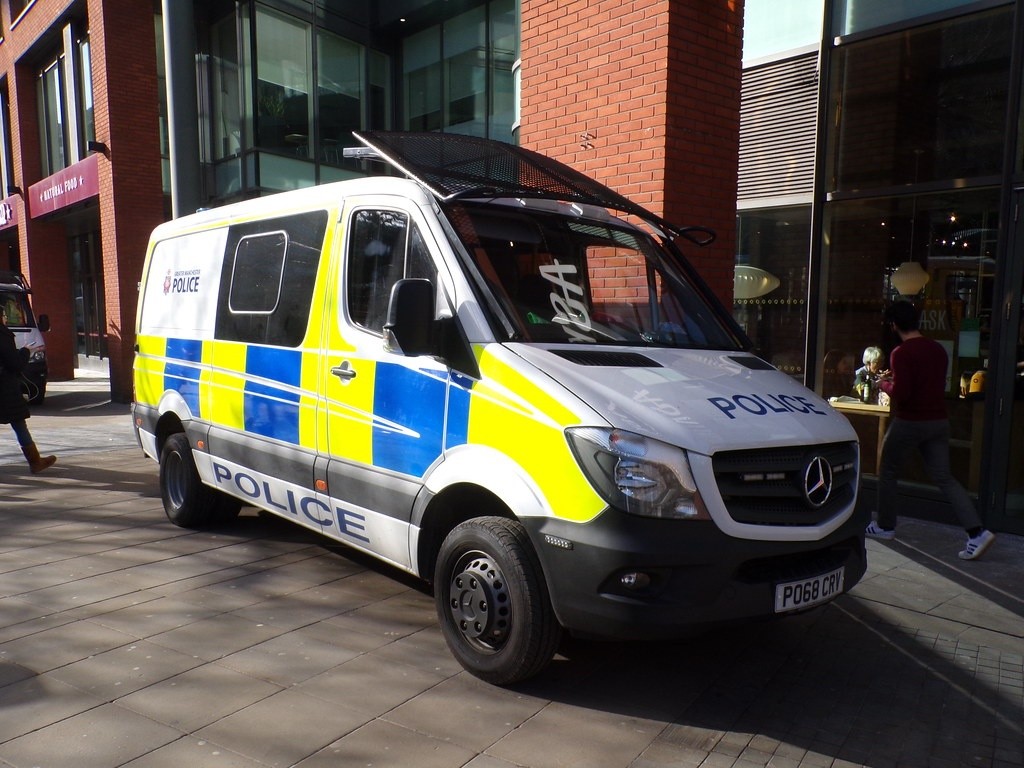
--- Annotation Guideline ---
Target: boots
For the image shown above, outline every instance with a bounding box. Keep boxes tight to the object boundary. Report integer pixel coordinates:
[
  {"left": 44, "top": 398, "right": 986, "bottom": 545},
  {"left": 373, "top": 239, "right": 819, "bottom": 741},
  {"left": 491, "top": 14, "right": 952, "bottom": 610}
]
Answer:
[{"left": 21, "top": 442, "right": 56, "bottom": 474}]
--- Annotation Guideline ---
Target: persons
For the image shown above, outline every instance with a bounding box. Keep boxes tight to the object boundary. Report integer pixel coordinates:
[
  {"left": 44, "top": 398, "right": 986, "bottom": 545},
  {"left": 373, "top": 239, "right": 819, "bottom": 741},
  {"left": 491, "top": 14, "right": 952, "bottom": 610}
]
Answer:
[
  {"left": 863, "top": 300, "right": 996, "bottom": 560},
  {"left": 0, "top": 305, "right": 57, "bottom": 475},
  {"left": 852, "top": 345, "right": 888, "bottom": 405}
]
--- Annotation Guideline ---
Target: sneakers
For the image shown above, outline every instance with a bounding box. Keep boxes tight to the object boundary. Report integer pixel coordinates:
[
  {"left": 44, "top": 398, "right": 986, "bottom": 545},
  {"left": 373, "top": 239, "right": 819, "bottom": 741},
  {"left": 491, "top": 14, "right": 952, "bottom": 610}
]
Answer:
[
  {"left": 864, "top": 519, "right": 896, "bottom": 540},
  {"left": 959, "top": 529, "right": 996, "bottom": 561}
]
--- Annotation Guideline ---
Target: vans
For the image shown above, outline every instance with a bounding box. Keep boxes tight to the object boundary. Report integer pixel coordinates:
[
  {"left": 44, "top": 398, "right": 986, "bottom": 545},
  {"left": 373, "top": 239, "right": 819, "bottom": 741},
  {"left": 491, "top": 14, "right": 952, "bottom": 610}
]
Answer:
[
  {"left": 0, "top": 272, "right": 51, "bottom": 406},
  {"left": 129, "top": 130, "right": 873, "bottom": 687}
]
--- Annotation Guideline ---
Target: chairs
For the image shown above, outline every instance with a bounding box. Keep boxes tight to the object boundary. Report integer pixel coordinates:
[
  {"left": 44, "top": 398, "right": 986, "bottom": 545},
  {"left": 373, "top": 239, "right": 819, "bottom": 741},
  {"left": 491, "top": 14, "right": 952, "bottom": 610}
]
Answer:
[{"left": 947, "top": 371, "right": 991, "bottom": 499}]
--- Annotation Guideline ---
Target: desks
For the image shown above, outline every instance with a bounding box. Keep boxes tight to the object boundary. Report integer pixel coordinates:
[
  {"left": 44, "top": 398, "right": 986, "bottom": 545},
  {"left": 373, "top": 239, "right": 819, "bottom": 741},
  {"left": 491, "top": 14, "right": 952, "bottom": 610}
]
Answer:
[{"left": 828, "top": 400, "right": 889, "bottom": 477}]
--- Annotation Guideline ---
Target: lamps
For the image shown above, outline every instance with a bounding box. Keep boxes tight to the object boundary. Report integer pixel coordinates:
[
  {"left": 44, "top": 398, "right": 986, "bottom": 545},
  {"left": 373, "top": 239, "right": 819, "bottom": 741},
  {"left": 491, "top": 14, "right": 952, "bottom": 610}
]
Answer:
[
  {"left": 733, "top": 217, "right": 780, "bottom": 300},
  {"left": 890, "top": 151, "right": 930, "bottom": 295}
]
